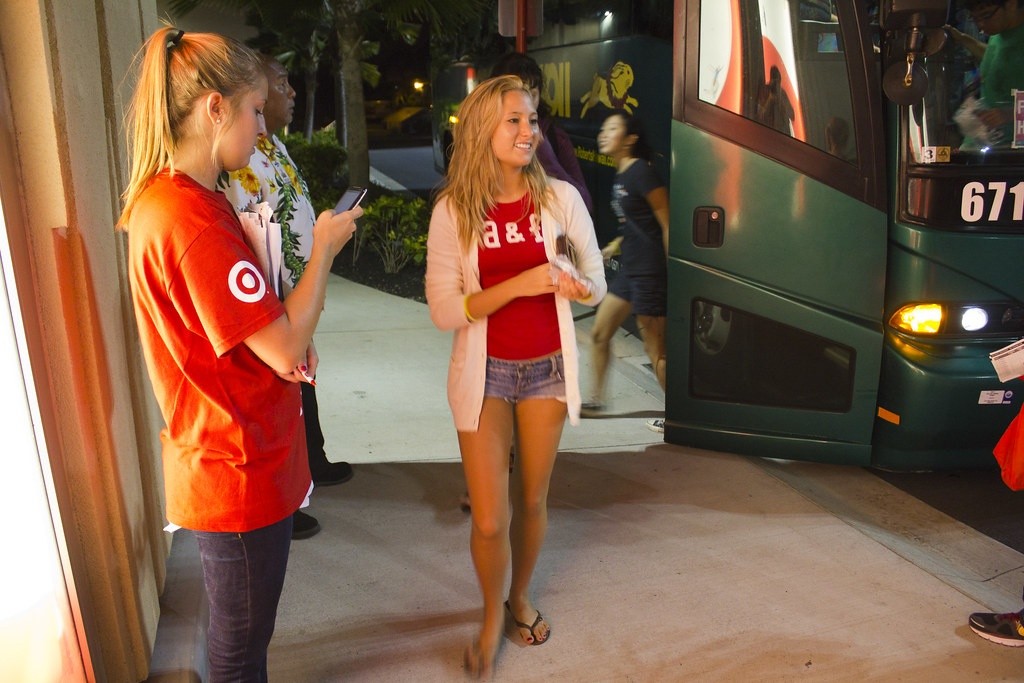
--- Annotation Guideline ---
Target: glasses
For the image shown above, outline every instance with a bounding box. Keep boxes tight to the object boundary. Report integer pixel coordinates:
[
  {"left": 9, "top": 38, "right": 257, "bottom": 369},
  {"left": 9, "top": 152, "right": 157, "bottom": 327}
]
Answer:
[{"left": 966, "top": 6, "right": 1000, "bottom": 25}]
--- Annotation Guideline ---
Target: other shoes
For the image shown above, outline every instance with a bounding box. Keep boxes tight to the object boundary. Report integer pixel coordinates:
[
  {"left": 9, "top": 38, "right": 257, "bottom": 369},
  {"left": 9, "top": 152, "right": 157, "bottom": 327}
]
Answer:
[
  {"left": 314, "top": 461, "right": 352, "bottom": 485},
  {"left": 290, "top": 509, "right": 319, "bottom": 541},
  {"left": 580, "top": 400, "right": 606, "bottom": 418},
  {"left": 646, "top": 418, "right": 665, "bottom": 434}
]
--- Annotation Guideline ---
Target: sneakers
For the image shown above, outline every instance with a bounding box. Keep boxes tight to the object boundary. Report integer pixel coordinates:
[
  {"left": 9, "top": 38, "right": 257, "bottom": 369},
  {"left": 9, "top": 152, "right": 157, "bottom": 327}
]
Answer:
[{"left": 968, "top": 608, "right": 1024, "bottom": 647}]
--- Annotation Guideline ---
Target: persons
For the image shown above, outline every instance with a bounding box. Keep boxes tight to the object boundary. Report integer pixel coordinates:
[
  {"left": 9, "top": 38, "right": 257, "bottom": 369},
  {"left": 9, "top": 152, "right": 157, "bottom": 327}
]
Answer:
[
  {"left": 426, "top": 75, "right": 607, "bottom": 683},
  {"left": 580, "top": 116, "right": 669, "bottom": 436},
  {"left": 115, "top": 24, "right": 362, "bottom": 683},
  {"left": 968, "top": 403, "right": 1024, "bottom": 647},
  {"left": 942, "top": 0, "right": 1024, "bottom": 150},
  {"left": 756, "top": 67, "right": 795, "bottom": 137},
  {"left": 498, "top": 52, "right": 594, "bottom": 213},
  {"left": 215, "top": 56, "right": 353, "bottom": 539},
  {"left": 826, "top": 117, "right": 850, "bottom": 156}
]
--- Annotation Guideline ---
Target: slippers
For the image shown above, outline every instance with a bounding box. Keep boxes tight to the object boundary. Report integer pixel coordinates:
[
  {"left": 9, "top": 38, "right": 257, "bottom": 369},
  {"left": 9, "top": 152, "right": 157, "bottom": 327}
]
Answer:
[{"left": 504, "top": 598, "right": 551, "bottom": 645}]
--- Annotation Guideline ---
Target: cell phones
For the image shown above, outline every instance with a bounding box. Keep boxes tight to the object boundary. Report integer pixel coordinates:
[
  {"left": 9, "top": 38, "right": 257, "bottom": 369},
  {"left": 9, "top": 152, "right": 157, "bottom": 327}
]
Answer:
[{"left": 334, "top": 186, "right": 367, "bottom": 214}]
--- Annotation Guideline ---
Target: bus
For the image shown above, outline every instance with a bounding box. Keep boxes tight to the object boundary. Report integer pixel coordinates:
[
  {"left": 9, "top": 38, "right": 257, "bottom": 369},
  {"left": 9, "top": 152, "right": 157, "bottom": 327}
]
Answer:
[{"left": 427, "top": 0, "right": 1024, "bottom": 479}]
